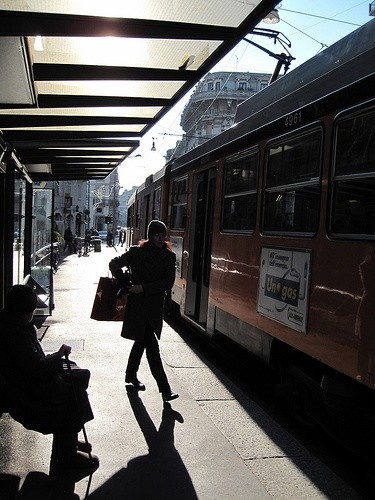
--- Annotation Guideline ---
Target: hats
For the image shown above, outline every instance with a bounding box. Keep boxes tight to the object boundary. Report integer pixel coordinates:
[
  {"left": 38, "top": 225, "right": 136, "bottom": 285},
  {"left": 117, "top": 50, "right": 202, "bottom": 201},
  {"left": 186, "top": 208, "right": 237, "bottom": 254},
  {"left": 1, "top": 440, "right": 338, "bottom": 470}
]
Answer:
[
  {"left": 148, "top": 220, "right": 167, "bottom": 239},
  {"left": 4, "top": 285, "right": 38, "bottom": 315}
]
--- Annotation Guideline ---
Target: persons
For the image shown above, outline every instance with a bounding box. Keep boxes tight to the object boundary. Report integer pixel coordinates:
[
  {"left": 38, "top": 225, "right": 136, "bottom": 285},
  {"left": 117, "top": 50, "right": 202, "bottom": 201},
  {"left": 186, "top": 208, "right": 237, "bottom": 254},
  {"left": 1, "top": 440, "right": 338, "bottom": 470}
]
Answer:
[
  {"left": 109, "top": 220, "right": 179, "bottom": 401},
  {"left": 87, "top": 230, "right": 99, "bottom": 236},
  {"left": 0, "top": 285, "right": 99, "bottom": 468},
  {"left": 117, "top": 231, "right": 126, "bottom": 247},
  {"left": 64, "top": 227, "right": 72, "bottom": 251}
]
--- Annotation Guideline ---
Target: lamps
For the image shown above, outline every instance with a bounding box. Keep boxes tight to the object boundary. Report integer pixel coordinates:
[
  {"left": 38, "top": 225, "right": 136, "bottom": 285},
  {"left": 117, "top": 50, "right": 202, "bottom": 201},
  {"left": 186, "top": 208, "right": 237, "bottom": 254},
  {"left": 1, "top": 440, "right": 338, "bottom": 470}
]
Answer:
[
  {"left": 150, "top": 137, "right": 157, "bottom": 152},
  {"left": 263, "top": 7, "right": 280, "bottom": 25}
]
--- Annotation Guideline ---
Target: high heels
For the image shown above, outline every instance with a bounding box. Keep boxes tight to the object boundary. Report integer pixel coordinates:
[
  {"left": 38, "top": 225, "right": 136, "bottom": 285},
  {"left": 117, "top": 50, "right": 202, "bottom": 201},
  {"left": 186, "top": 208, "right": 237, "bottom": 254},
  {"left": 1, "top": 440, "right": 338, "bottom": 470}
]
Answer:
[
  {"left": 125, "top": 373, "right": 145, "bottom": 389},
  {"left": 162, "top": 390, "right": 178, "bottom": 401}
]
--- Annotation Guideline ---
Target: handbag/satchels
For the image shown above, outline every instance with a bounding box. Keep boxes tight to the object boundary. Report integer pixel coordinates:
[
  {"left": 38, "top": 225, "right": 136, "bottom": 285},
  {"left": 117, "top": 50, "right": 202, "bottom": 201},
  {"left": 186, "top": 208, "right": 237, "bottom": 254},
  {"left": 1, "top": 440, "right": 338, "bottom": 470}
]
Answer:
[
  {"left": 90, "top": 268, "right": 128, "bottom": 321},
  {"left": 48, "top": 355, "right": 91, "bottom": 389}
]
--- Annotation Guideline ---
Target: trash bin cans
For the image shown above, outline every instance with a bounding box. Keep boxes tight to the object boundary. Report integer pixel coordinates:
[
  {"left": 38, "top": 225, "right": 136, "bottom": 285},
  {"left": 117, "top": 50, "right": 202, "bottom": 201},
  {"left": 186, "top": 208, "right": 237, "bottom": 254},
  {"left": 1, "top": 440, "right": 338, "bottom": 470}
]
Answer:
[{"left": 94, "top": 239, "right": 101, "bottom": 252}]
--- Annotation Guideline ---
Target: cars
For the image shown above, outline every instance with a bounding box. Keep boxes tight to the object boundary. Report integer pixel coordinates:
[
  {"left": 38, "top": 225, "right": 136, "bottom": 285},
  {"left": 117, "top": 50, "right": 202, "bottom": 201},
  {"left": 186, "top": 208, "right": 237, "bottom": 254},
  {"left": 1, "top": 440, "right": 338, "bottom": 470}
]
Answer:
[{"left": 99, "top": 231, "right": 108, "bottom": 240}]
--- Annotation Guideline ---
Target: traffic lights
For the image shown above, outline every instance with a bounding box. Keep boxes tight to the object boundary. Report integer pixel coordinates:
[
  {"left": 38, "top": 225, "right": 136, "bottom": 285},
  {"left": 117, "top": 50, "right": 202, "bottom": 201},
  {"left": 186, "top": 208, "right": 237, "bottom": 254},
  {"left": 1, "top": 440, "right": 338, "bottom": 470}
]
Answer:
[{"left": 110, "top": 216, "right": 113, "bottom": 223}]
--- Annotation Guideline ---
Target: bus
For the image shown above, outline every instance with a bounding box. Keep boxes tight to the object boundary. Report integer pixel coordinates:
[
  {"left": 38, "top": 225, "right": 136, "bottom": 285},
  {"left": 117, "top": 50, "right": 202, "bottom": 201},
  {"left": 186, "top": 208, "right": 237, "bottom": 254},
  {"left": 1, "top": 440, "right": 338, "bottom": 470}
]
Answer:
[{"left": 126, "top": 0, "right": 374, "bottom": 396}]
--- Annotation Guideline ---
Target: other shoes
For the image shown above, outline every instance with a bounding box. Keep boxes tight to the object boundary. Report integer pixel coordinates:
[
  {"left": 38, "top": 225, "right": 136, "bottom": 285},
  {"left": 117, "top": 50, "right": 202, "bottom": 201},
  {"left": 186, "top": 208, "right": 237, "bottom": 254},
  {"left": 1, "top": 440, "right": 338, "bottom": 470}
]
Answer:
[
  {"left": 72, "top": 453, "right": 98, "bottom": 470},
  {"left": 76, "top": 440, "right": 92, "bottom": 452}
]
